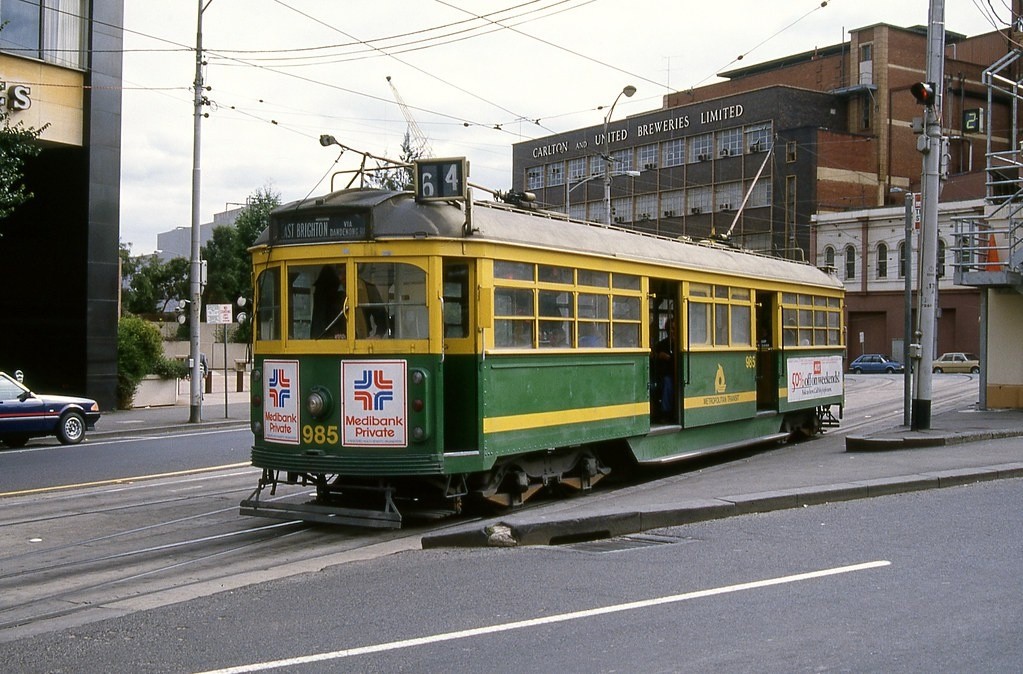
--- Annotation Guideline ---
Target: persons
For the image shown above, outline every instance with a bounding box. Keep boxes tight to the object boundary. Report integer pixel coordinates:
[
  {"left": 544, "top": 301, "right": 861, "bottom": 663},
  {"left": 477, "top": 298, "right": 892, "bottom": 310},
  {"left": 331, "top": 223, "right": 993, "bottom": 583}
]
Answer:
[
  {"left": 579, "top": 316, "right": 605, "bottom": 346},
  {"left": 326, "top": 263, "right": 388, "bottom": 338},
  {"left": 186, "top": 352, "right": 208, "bottom": 401},
  {"left": 658, "top": 318, "right": 679, "bottom": 425}
]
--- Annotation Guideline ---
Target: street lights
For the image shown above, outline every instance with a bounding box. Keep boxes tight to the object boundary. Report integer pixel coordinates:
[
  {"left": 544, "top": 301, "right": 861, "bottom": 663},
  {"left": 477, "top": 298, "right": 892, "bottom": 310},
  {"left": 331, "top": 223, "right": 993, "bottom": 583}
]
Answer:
[
  {"left": 604, "top": 85, "right": 637, "bottom": 226},
  {"left": 890, "top": 186, "right": 914, "bottom": 428},
  {"left": 565, "top": 170, "right": 640, "bottom": 223}
]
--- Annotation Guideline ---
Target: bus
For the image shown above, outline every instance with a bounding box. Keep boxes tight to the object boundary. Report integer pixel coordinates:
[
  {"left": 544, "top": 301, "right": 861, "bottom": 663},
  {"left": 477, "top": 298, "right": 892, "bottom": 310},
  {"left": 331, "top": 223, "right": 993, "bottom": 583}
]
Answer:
[{"left": 237, "top": 134, "right": 848, "bottom": 530}]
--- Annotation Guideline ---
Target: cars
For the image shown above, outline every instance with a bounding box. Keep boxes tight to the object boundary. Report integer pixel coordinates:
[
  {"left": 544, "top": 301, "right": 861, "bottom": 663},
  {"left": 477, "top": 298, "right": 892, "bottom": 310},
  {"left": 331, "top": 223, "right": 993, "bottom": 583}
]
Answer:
[
  {"left": 932, "top": 352, "right": 980, "bottom": 375},
  {"left": 849, "top": 352, "right": 913, "bottom": 375},
  {"left": 0, "top": 371, "right": 100, "bottom": 449}
]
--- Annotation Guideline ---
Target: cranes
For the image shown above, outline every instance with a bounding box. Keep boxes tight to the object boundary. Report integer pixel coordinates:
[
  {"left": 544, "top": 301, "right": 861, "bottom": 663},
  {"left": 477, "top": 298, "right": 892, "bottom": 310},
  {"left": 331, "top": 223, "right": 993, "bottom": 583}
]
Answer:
[{"left": 386, "top": 75, "right": 437, "bottom": 158}]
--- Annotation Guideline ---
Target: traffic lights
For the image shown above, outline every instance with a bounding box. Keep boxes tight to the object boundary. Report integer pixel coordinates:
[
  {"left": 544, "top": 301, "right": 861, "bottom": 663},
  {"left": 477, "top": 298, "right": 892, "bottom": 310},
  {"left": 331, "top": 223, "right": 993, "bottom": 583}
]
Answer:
[{"left": 910, "top": 81, "right": 936, "bottom": 106}]
